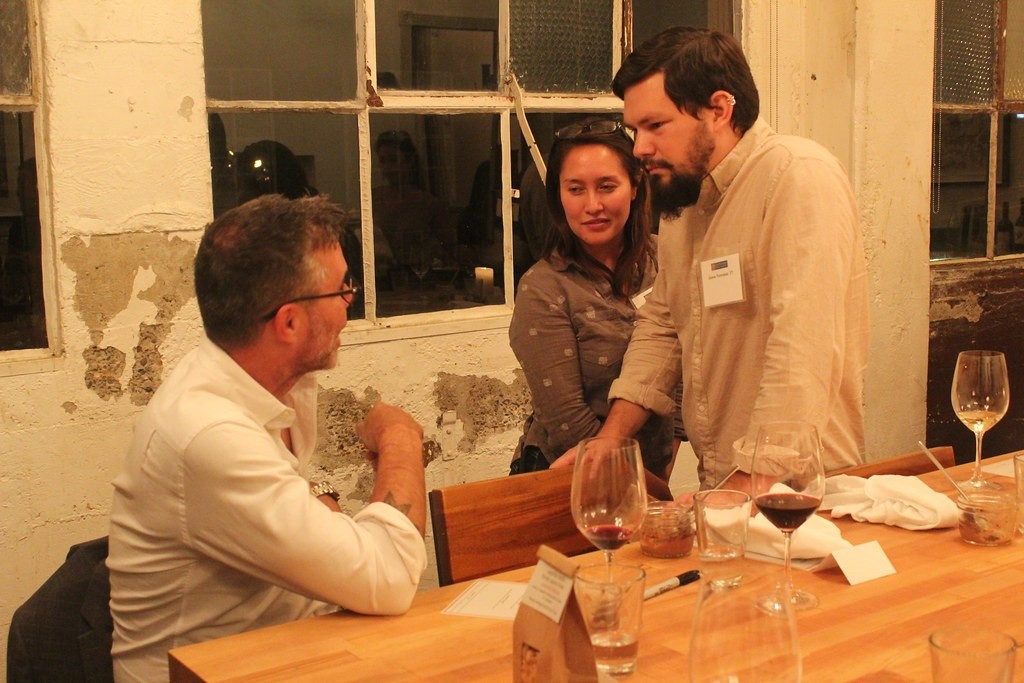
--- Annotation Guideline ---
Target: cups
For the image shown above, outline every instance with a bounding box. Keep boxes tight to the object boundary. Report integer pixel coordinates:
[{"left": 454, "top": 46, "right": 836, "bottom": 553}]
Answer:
[
  {"left": 928, "top": 626, "right": 1017, "bottom": 683},
  {"left": 640, "top": 501, "right": 695, "bottom": 562},
  {"left": 571, "top": 563, "right": 646, "bottom": 672},
  {"left": 957, "top": 491, "right": 1017, "bottom": 547},
  {"left": 1014, "top": 454, "right": 1024, "bottom": 535},
  {"left": 690, "top": 572, "right": 800, "bottom": 683},
  {"left": 690, "top": 490, "right": 750, "bottom": 587}
]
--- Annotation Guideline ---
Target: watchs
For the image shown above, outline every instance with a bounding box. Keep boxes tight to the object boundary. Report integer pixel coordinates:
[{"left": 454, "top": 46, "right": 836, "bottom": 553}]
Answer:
[{"left": 309, "top": 481, "right": 342, "bottom": 501}]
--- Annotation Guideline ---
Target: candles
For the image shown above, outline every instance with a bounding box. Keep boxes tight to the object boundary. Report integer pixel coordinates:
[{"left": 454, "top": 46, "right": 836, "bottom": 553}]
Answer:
[{"left": 476, "top": 265, "right": 494, "bottom": 303}]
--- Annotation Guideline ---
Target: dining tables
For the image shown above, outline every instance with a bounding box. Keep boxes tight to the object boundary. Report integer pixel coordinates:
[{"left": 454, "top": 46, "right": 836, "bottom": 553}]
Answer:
[{"left": 167, "top": 448, "right": 1024, "bottom": 683}]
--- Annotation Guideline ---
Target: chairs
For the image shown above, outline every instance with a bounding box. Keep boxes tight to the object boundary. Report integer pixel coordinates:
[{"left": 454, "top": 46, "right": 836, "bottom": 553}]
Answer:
[
  {"left": 824, "top": 446, "right": 956, "bottom": 476},
  {"left": 428, "top": 464, "right": 600, "bottom": 587}
]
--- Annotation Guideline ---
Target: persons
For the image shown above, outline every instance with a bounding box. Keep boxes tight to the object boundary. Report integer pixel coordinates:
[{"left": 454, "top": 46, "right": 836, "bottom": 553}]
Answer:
[
  {"left": 506, "top": 115, "right": 689, "bottom": 505},
  {"left": 549, "top": 25, "right": 869, "bottom": 516},
  {"left": 103, "top": 192, "right": 428, "bottom": 683},
  {"left": 206, "top": 110, "right": 538, "bottom": 322}
]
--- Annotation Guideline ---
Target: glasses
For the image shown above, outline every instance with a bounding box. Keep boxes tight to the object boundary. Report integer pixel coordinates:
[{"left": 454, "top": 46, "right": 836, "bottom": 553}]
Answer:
[
  {"left": 261, "top": 271, "right": 361, "bottom": 323},
  {"left": 555, "top": 118, "right": 627, "bottom": 139}
]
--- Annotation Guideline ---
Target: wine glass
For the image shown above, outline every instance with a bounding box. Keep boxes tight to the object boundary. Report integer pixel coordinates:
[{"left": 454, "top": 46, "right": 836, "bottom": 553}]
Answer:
[
  {"left": 750, "top": 421, "right": 826, "bottom": 615},
  {"left": 568, "top": 435, "right": 649, "bottom": 582},
  {"left": 950, "top": 350, "right": 1010, "bottom": 493}
]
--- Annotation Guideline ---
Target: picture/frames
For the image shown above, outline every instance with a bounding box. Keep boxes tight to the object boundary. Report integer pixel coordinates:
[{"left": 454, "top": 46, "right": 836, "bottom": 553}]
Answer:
[{"left": 931, "top": 110, "right": 1009, "bottom": 188}]
